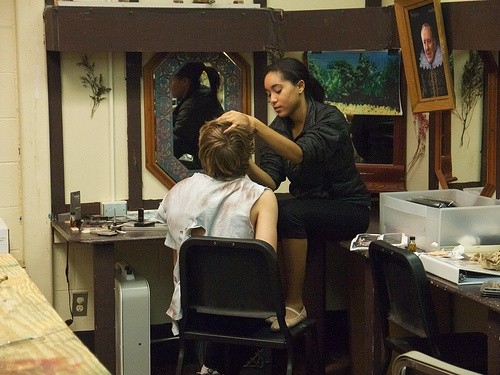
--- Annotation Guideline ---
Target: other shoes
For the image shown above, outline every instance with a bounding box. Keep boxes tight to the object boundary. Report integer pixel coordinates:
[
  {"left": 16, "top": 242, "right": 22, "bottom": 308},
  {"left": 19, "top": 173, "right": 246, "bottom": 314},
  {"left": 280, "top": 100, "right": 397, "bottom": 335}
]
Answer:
[{"left": 271, "top": 306, "right": 307, "bottom": 330}]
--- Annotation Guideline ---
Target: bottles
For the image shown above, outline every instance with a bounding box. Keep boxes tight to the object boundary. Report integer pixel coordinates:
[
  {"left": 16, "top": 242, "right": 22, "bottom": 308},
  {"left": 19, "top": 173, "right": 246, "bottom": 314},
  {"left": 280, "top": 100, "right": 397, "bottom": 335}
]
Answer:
[
  {"left": 409, "top": 237, "right": 416, "bottom": 252},
  {"left": 70, "top": 212, "right": 76, "bottom": 227}
]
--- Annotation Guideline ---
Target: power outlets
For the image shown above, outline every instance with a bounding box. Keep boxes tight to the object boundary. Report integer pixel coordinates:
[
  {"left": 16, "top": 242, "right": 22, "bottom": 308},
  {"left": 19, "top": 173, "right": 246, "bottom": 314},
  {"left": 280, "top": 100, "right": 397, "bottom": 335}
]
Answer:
[{"left": 72, "top": 291, "right": 88, "bottom": 316}]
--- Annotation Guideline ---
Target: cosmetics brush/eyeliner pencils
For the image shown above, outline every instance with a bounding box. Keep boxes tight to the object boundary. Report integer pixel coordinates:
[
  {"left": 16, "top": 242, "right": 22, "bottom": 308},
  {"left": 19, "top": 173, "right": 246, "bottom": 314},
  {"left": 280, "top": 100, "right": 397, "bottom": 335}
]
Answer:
[{"left": 113, "top": 208, "right": 117, "bottom": 225}]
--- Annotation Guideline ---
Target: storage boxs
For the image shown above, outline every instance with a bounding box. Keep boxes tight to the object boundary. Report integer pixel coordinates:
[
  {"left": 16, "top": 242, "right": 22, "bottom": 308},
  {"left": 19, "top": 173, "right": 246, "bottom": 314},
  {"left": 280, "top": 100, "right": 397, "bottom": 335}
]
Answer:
[{"left": 379, "top": 189, "right": 499, "bottom": 253}]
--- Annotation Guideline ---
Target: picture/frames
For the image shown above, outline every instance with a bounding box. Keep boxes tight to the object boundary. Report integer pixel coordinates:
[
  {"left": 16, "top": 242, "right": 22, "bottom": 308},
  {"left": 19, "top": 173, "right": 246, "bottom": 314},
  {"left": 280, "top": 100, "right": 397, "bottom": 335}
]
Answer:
[{"left": 395, "top": 0, "right": 456, "bottom": 114}]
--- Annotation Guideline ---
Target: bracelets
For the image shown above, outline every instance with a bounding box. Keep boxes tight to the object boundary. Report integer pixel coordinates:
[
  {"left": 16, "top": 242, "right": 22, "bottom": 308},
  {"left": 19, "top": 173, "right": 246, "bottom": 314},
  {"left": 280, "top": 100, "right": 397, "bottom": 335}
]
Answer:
[{"left": 248, "top": 115, "right": 254, "bottom": 130}]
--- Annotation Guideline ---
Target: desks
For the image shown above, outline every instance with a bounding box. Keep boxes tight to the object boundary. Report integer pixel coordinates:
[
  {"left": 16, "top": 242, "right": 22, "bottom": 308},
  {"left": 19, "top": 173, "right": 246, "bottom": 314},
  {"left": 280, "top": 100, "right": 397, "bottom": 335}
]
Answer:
[
  {"left": 339, "top": 235, "right": 500, "bottom": 375},
  {"left": 0, "top": 254, "right": 113, "bottom": 375},
  {"left": 50, "top": 214, "right": 170, "bottom": 375}
]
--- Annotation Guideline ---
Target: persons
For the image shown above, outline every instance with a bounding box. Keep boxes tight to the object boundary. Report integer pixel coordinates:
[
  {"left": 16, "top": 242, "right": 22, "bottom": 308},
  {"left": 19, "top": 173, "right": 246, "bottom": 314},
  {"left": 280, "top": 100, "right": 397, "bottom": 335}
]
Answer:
[
  {"left": 172, "top": 61, "right": 225, "bottom": 170},
  {"left": 419, "top": 23, "right": 448, "bottom": 98},
  {"left": 157, "top": 115, "right": 304, "bottom": 375},
  {"left": 212, "top": 57, "right": 371, "bottom": 332}
]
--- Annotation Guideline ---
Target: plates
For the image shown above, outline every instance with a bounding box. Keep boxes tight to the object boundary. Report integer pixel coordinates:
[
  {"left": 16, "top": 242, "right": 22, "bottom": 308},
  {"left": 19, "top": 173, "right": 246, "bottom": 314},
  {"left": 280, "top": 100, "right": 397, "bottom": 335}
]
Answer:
[{"left": 480, "top": 281, "right": 500, "bottom": 297}]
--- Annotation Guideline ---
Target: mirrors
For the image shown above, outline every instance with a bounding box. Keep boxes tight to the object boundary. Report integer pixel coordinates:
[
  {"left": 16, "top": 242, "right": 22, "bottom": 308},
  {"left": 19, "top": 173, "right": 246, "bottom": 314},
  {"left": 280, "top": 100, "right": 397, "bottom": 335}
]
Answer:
[{"left": 143, "top": 52, "right": 251, "bottom": 192}]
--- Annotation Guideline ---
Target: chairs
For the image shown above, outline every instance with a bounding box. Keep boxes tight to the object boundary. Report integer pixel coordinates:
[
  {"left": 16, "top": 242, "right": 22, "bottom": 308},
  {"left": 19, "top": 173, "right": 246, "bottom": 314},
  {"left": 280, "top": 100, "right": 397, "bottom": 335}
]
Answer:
[
  {"left": 176, "top": 236, "right": 324, "bottom": 375},
  {"left": 369, "top": 240, "right": 488, "bottom": 375}
]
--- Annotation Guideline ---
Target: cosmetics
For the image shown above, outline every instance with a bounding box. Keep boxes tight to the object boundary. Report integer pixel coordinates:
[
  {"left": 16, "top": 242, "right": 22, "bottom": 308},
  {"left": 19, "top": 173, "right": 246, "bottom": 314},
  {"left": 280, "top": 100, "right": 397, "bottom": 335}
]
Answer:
[
  {"left": 408, "top": 236, "right": 416, "bottom": 254},
  {"left": 138, "top": 208, "right": 145, "bottom": 222},
  {"left": 70, "top": 190, "right": 82, "bottom": 227}
]
